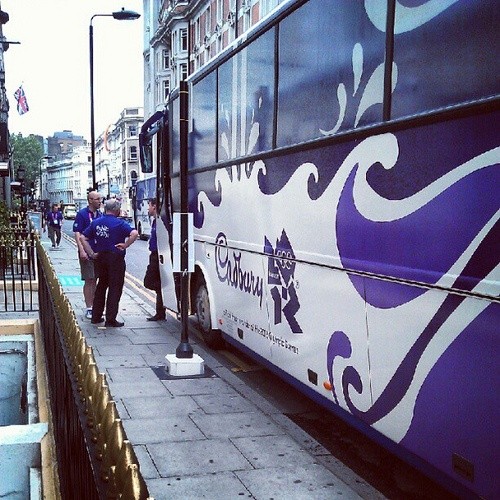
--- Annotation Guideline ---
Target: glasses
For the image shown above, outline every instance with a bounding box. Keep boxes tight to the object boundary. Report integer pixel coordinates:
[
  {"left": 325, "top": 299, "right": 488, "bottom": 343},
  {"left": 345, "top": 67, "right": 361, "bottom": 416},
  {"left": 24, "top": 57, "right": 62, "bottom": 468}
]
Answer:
[{"left": 90, "top": 197, "right": 100, "bottom": 200}]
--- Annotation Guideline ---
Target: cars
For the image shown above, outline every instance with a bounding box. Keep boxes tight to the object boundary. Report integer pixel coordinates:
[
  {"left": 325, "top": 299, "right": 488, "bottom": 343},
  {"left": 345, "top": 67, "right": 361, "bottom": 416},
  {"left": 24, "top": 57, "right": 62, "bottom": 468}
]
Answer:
[{"left": 64, "top": 209, "right": 77, "bottom": 219}]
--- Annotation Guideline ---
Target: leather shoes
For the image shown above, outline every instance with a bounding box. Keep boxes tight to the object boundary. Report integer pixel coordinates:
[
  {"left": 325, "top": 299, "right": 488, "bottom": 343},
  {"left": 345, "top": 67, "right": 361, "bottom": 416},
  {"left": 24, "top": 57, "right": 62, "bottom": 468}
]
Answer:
[
  {"left": 146, "top": 313, "right": 166, "bottom": 321},
  {"left": 104, "top": 320, "right": 124, "bottom": 327},
  {"left": 91, "top": 318, "right": 104, "bottom": 324}
]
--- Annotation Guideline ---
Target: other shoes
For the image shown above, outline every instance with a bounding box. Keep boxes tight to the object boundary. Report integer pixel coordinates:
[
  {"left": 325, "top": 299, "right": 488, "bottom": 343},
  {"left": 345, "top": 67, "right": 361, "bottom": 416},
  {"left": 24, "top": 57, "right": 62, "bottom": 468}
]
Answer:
[
  {"left": 57, "top": 244, "right": 59, "bottom": 247},
  {"left": 52, "top": 245, "right": 55, "bottom": 248}
]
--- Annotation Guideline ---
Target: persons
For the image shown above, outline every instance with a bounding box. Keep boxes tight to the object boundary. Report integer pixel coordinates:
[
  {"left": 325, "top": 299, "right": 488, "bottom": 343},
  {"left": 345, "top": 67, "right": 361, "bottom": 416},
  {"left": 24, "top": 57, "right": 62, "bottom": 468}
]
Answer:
[
  {"left": 46, "top": 203, "right": 65, "bottom": 247},
  {"left": 143, "top": 198, "right": 167, "bottom": 322},
  {"left": 72, "top": 189, "right": 104, "bottom": 319},
  {"left": 79, "top": 198, "right": 139, "bottom": 327}
]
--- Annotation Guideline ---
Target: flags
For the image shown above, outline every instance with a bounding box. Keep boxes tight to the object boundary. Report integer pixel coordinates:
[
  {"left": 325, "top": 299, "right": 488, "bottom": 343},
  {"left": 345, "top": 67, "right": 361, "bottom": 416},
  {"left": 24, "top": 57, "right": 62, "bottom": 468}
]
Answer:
[{"left": 15, "top": 84, "right": 30, "bottom": 116}]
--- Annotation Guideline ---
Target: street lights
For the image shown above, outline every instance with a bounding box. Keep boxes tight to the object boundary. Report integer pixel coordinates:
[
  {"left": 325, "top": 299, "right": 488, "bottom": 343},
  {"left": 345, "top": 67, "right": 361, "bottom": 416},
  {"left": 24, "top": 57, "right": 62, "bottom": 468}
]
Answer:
[
  {"left": 89, "top": 7, "right": 141, "bottom": 192},
  {"left": 37, "top": 154, "right": 55, "bottom": 198}
]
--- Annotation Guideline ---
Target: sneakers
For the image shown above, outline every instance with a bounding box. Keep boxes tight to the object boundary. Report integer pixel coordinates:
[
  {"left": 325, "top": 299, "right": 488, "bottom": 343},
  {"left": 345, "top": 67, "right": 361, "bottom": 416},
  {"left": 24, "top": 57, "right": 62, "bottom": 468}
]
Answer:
[{"left": 86, "top": 310, "right": 92, "bottom": 318}]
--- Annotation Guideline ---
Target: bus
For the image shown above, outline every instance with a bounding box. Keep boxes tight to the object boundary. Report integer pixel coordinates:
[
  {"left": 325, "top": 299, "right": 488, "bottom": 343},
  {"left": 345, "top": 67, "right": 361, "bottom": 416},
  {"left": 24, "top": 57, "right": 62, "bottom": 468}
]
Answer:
[{"left": 138, "top": 1, "right": 499, "bottom": 482}]
